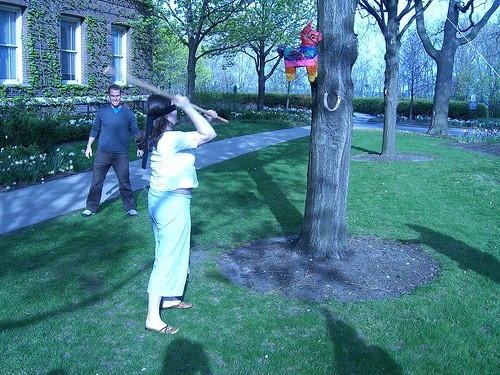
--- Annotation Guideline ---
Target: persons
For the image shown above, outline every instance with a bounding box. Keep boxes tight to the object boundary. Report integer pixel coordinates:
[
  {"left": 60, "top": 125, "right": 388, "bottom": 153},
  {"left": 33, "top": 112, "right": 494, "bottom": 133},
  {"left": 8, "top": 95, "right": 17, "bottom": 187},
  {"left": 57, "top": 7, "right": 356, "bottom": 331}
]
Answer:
[
  {"left": 81, "top": 84, "right": 145, "bottom": 217},
  {"left": 141, "top": 93, "right": 218, "bottom": 335}
]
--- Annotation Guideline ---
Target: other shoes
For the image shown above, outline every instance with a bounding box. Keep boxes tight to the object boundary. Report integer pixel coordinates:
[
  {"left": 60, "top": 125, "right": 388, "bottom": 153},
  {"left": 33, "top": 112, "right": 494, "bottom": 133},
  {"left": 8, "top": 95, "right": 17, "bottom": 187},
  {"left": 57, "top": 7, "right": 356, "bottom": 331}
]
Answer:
[
  {"left": 125, "top": 209, "right": 138, "bottom": 216},
  {"left": 81, "top": 209, "right": 96, "bottom": 216}
]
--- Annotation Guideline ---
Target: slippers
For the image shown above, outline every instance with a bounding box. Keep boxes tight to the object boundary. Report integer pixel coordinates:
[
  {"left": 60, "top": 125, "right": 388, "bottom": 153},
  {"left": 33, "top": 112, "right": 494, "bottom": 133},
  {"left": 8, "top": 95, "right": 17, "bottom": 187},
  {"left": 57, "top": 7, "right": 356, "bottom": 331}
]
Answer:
[
  {"left": 145, "top": 324, "right": 180, "bottom": 335},
  {"left": 161, "top": 299, "right": 192, "bottom": 310}
]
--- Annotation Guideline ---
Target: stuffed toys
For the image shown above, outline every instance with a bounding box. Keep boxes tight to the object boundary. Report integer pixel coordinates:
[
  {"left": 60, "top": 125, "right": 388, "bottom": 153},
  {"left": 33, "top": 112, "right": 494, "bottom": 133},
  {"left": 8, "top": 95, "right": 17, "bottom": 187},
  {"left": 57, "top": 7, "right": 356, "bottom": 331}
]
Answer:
[{"left": 277, "top": 20, "right": 322, "bottom": 86}]
